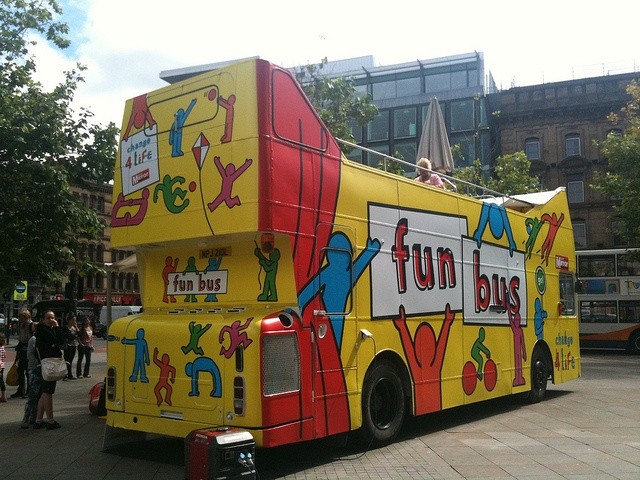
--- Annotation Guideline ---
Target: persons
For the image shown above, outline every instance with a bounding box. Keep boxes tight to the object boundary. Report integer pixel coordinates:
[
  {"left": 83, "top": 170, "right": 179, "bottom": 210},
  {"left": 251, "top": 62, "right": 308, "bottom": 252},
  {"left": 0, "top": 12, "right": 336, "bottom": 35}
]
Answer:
[
  {"left": 0, "top": 334, "right": 9, "bottom": 402},
  {"left": 11, "top": 310, "right": 37, "bottom": 398},
  {"left": 63, "top": 315, "right": 80, "bottom": 381},
  {"left": 21, "top": 333, "right": 43, "bottom": 428},
  {"left": 414, "top": 157, "right": 445, "bottom": 189},
  {"left": 34, "top": 310, "right": 65, "bottom": 431},
  {"left": 77, "top": 316, "right": 95, "bottom": 378}
]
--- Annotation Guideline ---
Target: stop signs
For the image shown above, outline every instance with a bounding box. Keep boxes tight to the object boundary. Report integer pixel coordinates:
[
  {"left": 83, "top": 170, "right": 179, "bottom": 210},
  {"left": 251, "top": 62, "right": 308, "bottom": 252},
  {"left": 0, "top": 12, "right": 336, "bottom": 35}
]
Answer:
[{"left": 14, "top": 280, "right": 28, "bottom": 301}]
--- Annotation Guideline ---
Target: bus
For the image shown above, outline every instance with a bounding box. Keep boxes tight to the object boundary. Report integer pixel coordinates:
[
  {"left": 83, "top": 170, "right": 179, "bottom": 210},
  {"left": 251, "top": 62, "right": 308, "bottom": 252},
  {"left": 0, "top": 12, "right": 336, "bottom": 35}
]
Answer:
[
  {"left": 573, "top": 249, "right": 640, "bottom": 350},
  {"left": 106, "top": 58, "right": 583, "bottom": 451}
]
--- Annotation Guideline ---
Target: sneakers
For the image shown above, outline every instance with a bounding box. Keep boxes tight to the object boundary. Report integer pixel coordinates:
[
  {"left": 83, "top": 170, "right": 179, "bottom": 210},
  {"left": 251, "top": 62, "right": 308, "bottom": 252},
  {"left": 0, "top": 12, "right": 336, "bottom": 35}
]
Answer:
[
  {"left": 33, "top": 421, "right": 47, "bottom": 429},
  {"left": 45, "top": 421, "right": 61, "bottom": 430}
]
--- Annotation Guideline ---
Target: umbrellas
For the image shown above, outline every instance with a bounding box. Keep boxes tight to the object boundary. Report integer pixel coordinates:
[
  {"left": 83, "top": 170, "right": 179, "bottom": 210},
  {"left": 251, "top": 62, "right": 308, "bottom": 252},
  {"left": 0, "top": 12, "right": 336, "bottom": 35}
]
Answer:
[{"left": 416, "top": 96, "right": 454, "bottom": 171}]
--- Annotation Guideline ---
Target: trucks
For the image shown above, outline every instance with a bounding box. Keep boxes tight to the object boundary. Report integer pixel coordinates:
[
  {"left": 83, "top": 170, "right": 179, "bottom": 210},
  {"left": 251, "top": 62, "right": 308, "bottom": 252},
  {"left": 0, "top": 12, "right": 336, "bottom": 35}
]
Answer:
[{"left": 100, "top": 305, "right": 142, "bottom": 340}]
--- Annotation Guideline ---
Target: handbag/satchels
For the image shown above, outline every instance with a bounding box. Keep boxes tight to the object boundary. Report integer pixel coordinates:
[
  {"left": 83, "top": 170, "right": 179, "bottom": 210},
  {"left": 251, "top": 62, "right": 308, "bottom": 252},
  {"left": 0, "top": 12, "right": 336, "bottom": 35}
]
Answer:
[
  {"left": 36, "top": 346, "right": 71, "bottom": 382},
  {"left": 6, "top": 357, "right": 19, "bottom": 386}
]
